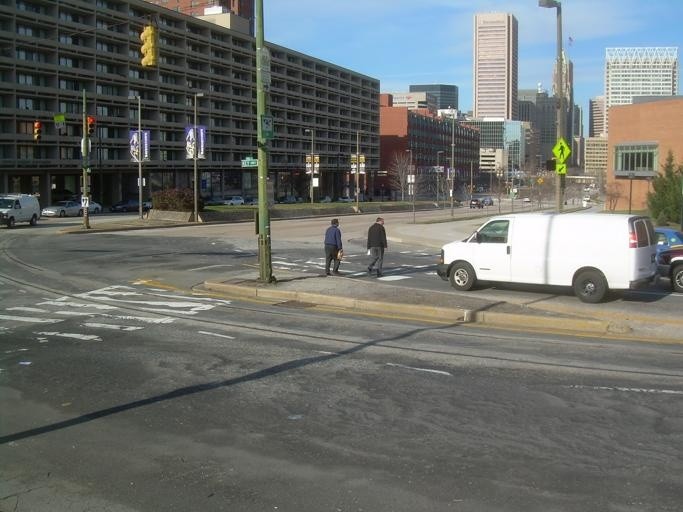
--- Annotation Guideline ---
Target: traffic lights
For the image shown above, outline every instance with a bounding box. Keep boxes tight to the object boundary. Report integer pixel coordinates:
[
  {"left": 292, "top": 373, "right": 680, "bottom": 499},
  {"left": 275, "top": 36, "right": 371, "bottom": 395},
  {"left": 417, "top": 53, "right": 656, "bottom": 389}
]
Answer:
[
  {"left": 33, "top": 121, "right": 41, "bottom": 142},
  {"left": 87, "top": 116, "right": 95, "bottom": 136},
  {"left": 140, "top": 26, "right": 157, "bottom": 66}
]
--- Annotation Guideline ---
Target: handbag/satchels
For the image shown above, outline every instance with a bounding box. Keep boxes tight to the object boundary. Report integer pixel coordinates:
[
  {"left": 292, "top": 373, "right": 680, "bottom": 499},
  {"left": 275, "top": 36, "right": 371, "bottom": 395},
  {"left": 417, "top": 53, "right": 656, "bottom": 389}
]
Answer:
[
  {"left": 367, "top": 248, "right": 371, "bottom": 256},
  {"left": 337, "top": 250, "right": 343, "bottom": 261}
]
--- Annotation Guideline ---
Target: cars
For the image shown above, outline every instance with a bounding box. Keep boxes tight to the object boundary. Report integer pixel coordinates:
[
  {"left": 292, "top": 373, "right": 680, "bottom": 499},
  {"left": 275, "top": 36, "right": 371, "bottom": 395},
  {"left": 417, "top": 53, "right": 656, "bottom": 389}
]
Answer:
[
  {"left": 42, "top": 201, "right": 102, "bottom": 217},
  {"left": 204, "top": 196, "right": 257, "bottom": 205},
  {"left": 112, "top": 198, "right": 153, "bottom": 212},
  {"left": 470, "top": 197, "right": 493, "bottom": 208},
  {"left": 655, "top": 228, "right": 683, "bottom": 293},
  {"left": 316, "top": 196, "right": 331, "bottom": 202}
]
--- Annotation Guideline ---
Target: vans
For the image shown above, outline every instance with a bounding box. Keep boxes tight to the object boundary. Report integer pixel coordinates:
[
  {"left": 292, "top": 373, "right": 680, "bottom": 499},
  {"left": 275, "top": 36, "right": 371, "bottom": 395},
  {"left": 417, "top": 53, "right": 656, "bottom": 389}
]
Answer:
[
  {"left": 437, "top": 212, "right": 656, "bottom": 303},
  {"left": 0, "top": 193, "right": 40, "bottom": 228}
]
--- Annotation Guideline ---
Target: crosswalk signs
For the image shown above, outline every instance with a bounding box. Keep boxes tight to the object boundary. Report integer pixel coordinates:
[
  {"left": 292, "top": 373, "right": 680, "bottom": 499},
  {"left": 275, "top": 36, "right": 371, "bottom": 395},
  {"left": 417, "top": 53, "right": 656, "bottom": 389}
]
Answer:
[{"left": 553, "top": 139, "right": 570, "bottom": 163}]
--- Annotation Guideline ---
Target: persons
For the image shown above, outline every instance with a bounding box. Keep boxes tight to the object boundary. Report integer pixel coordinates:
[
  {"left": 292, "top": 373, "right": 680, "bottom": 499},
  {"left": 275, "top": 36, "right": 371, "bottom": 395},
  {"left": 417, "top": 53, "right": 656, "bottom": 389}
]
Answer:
[
  {"left": 325, "top": 219, "right": 343, "bottom": 275},
  {"left": 368, "top": 218, "right": 387, "bottom": 277}
]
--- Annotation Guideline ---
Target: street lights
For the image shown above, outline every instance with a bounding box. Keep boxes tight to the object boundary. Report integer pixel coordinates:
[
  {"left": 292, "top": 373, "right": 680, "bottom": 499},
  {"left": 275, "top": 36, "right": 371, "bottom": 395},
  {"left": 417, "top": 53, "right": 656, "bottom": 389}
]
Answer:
[
  {"left": 538, "top": 0, "right": 565, "bottom": 210},
  {"left": 445, "top": 113, "right": 455, "bottom": 216},
  {"left": 128, "top": 95, "right": 142, "bottom": 215},
  {"left": 628, "top": 172, "right": 635, "bottom": 213},
  {"left": 193, "top": 92, "right": 204, "bottom": 221},
  {"left": 436, "top": 150, "right": 444, "bottom": 203},
  {"left": 304, "top": 128, "right": 314, "bottom": 203},
  {"left": 405, "top": 149, "right": 413, "bottom": 202}
]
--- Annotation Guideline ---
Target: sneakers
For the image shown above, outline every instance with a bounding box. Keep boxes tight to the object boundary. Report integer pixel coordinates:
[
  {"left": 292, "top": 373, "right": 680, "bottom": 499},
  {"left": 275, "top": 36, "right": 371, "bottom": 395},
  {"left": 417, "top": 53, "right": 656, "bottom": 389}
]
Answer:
[
  {"left": 368, "top": 268, "right": 385, "bottom": 277},
  {"left": 326, "top": 270, "right": 341, "bottom": 276}
]
